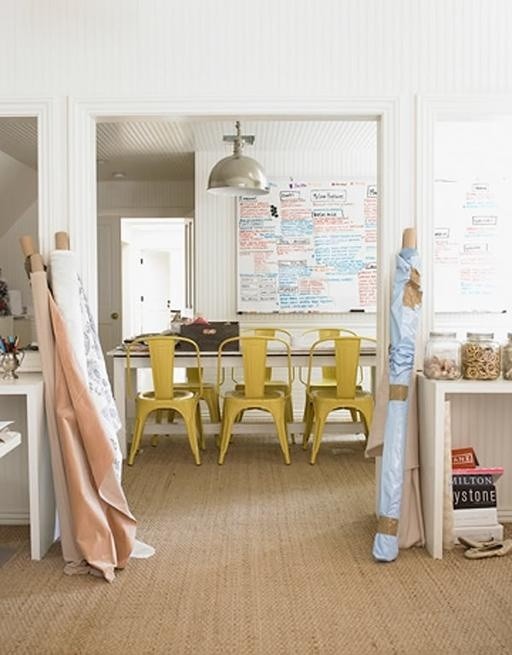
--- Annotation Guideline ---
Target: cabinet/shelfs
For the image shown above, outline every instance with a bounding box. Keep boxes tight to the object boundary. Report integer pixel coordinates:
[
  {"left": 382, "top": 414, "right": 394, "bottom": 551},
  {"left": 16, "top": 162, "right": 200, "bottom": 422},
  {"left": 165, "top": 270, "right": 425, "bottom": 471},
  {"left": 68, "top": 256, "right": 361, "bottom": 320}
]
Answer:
[
  {"left": 415, "top": 372, "right": 512, "bottom": 560},
  {"left": 0, "top": 372, "right": 61, "bottom": 561}
]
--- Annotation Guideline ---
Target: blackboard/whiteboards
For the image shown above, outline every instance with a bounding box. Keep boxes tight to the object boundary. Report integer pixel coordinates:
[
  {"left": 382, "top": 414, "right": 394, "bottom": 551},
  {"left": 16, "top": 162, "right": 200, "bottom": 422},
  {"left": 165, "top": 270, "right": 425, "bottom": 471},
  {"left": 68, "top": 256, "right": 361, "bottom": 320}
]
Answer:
[{"left": 236, "top": 179, "right": 509, "bottom": 314}]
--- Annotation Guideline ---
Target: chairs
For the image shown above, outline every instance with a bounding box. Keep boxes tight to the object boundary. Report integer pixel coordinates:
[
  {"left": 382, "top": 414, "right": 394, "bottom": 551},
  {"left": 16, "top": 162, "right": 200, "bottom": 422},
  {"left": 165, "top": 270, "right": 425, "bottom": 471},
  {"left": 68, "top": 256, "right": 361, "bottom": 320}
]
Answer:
[
  {"left": 214, "top": 337, "right": 296, "bottom": 465},
  {"left": 155, "top": 367, "right": 224, "bottom": 448},
  {"left": 124, "top": 335, "right": 206, "bottom": 467},
  {"left": 297, "top": 326, "right": 364, "bottom": 434},
  {"left": 302, "top": 334, "right": 377, "bottom": 466},
  {"left": 227, "top": 326, "right": 294, "bottom": 444}
]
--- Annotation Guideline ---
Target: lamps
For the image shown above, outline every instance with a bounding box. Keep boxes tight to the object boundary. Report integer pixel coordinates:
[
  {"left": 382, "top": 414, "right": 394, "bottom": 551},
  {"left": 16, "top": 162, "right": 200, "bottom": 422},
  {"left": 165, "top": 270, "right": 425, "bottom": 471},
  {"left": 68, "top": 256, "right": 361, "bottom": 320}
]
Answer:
[{"left": 207, "top": 121, "right": 270, "bottom": 198}]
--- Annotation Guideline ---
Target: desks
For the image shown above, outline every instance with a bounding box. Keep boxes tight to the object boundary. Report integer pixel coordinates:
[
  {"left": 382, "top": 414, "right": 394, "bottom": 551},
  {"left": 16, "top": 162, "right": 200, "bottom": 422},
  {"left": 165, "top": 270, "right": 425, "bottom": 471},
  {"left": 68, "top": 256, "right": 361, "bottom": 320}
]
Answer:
[{"left": 106, "top": 336, "right": 380, "bottom": 460}]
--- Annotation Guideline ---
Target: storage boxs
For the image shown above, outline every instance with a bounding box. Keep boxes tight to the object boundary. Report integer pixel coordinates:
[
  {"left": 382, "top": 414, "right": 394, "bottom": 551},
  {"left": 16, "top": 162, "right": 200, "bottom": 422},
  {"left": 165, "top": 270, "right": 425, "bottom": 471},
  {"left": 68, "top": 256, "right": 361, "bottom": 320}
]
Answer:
[{"left": 179, "top": 321, "right": 240, "bottom": 352}]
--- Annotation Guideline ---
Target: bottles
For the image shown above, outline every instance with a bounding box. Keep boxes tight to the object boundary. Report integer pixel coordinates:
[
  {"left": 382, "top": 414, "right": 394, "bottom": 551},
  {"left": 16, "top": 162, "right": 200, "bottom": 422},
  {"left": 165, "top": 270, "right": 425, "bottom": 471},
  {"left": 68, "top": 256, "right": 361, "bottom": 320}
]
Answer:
[
  {"left": 423, "top": 328, "right": 462, "bottom": 381},
  {"left": 459, "top": 333, "right": 500, "bottom": 381},
  {"left": 496, "top": 333, "right": 509, "bottom": 380}
]
